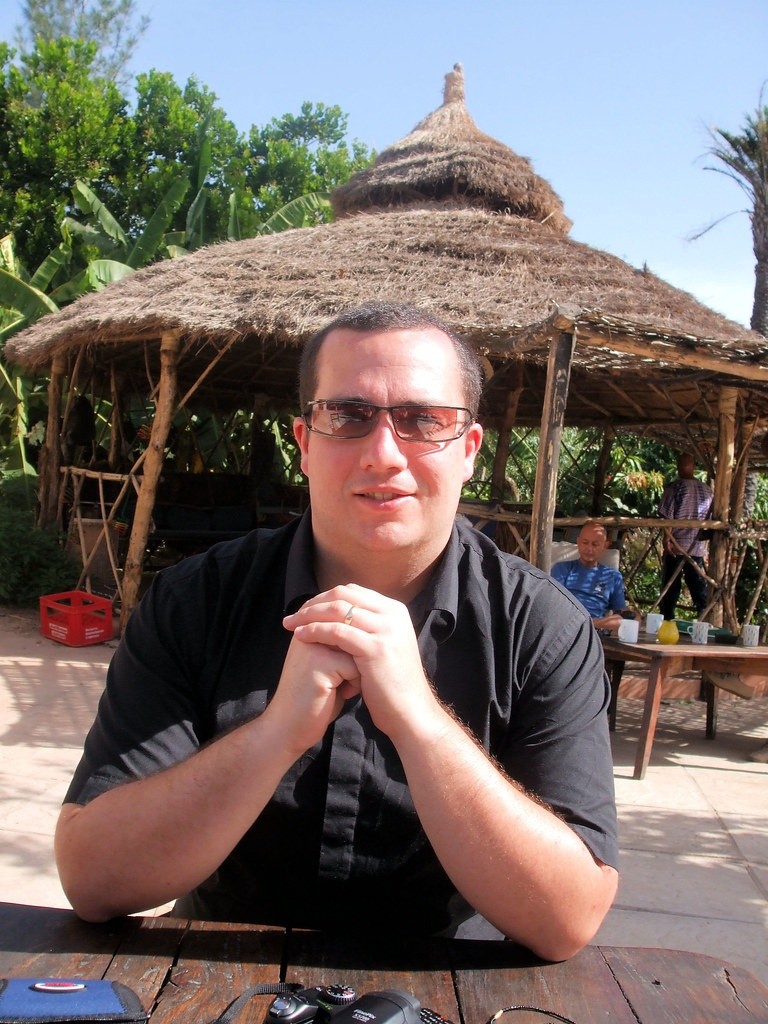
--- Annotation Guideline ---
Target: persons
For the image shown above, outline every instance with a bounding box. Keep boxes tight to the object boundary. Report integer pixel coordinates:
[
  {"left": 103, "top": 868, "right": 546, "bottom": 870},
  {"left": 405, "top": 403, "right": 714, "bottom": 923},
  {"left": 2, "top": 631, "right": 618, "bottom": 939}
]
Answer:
[
  {"left": 56, "top": 299, "right": 619, "bottom": 963},
  {"left": 551, "top": 520, "right": 628, "bottom": 638},
  {"left": 657, "top": 451, "right": 714, "bottom": 623}
]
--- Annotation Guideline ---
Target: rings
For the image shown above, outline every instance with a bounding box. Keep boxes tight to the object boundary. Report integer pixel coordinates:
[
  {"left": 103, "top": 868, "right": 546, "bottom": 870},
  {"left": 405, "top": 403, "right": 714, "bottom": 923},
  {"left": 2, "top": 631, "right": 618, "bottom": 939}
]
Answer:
[{"left": 344, "top": 605, "right": 357, "bottom": 625}]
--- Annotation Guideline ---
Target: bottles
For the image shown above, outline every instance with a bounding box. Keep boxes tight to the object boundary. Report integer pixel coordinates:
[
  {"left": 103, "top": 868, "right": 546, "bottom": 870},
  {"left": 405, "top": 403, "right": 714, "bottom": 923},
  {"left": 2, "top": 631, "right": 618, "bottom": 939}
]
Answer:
[{"left": 657, "top": 621, "right": 680, "bottom": 645}]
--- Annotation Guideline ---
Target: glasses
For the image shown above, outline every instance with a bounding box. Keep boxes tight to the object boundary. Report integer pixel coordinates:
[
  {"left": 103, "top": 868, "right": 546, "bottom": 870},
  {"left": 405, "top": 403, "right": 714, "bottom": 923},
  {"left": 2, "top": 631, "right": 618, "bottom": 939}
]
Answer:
[{"left": 302, "top": 398, "right": 476, "bottom": 443}]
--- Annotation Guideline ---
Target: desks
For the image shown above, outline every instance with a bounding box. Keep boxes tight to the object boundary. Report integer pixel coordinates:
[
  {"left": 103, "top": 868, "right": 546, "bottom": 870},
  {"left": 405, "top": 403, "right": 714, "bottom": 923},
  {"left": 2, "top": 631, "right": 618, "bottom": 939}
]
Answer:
[
  {"left": 0, "top": 901, "right": 768, "bottom": 1024},
  {"left": 600, "top": 632, "right": 768, "bottom": 780}
]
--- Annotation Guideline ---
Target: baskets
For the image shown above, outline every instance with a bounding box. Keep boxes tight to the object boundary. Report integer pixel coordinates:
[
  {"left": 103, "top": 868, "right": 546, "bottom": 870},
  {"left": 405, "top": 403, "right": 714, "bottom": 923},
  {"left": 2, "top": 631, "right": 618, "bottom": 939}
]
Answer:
[{"left": 40, "top": 590, "right": 114, "bottom": 647}]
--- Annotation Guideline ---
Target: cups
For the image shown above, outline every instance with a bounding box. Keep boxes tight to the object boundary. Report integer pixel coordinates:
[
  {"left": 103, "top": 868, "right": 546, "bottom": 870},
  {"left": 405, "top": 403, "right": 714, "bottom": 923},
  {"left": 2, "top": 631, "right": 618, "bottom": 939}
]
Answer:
[
  {"left": 645, "top": 613, "right": 665, "bottom": 636},
  {"left": 743, "top": 625, "right": 760, "bottom": 645},
  {"left": 616, "top": 619, "right": 640, "bottom": 643},
  {"left": 687, "top": 621, "right": 709, "bottom": 644}
]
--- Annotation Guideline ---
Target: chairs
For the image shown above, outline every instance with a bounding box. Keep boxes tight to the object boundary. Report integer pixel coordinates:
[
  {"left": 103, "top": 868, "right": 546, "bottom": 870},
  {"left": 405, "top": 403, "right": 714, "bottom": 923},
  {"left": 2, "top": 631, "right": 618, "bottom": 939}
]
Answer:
[{"left": 549, "top": 540, "right": 620, "bottom": 576}]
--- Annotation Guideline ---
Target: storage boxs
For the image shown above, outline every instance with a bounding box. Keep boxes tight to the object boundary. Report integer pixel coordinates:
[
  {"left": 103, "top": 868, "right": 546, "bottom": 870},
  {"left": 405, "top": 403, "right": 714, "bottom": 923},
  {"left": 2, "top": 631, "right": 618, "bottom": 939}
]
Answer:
[{"left": 39, "top": 590, "right": 115, "bottom": 647}]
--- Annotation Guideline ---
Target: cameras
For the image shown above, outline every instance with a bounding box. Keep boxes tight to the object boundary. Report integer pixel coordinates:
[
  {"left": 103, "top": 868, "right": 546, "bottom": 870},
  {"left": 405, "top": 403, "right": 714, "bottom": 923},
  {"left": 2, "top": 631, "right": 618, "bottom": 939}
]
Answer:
[{"left": 262, "top": 985, "right": 427, "bottom": 1024}]
